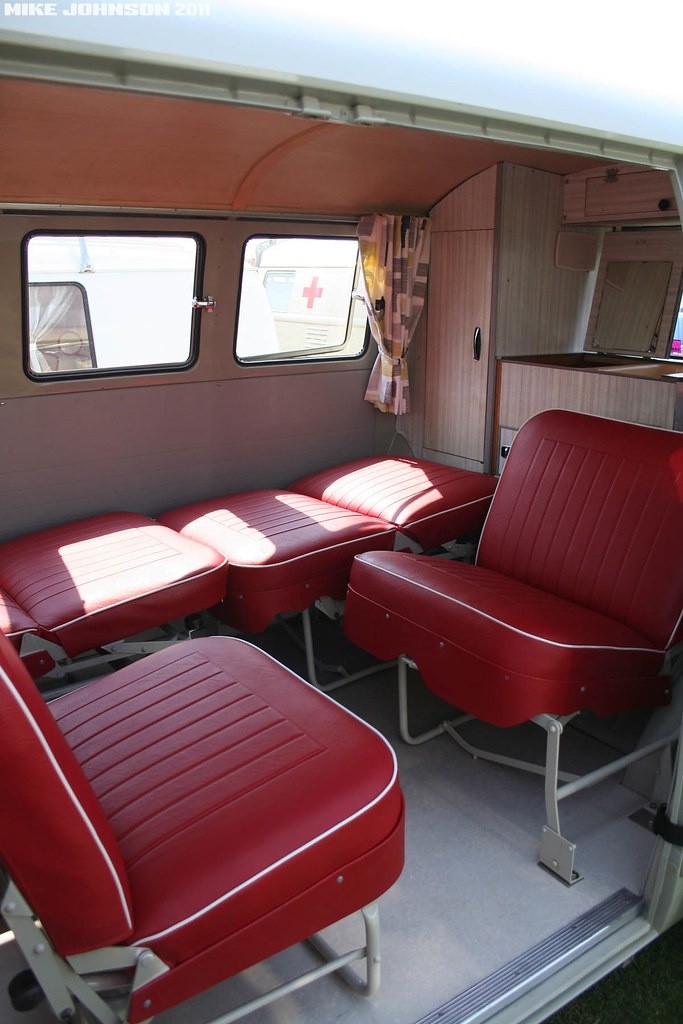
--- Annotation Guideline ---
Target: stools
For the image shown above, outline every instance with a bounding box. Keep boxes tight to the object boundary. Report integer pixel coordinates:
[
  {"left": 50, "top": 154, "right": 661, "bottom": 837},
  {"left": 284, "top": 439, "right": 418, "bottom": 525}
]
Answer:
[
  {"left": 289, "top": 455, "right": 498, "bottom": 552},
  {"left": 157, "top": 489, "right": 396, "bottom": 692}
]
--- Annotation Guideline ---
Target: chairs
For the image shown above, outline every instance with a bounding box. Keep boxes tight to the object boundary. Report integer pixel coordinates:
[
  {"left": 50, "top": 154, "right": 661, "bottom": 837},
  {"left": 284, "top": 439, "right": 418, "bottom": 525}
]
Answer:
[
  {"left": 0, "top": 636, "right": 405, "bottom": 1024},
  {"left": 0, "top": 510, "right": 228, "bottom": 678},
  {"left": 343, "top": 408, "right": 683, "bottom": 885}
]
